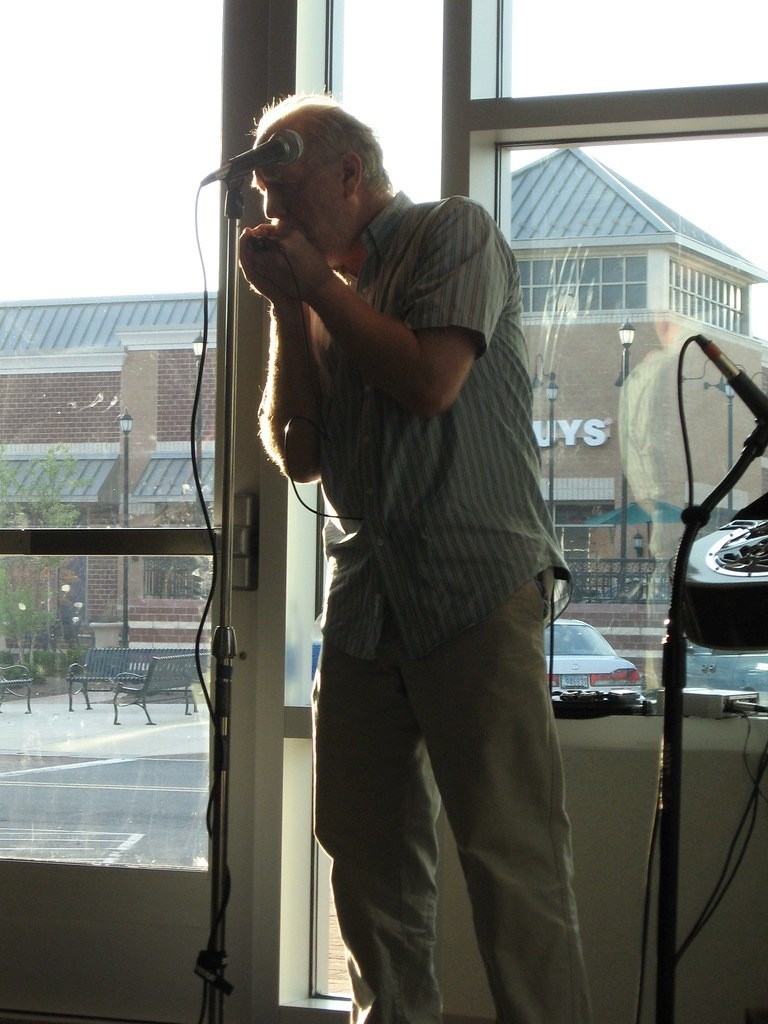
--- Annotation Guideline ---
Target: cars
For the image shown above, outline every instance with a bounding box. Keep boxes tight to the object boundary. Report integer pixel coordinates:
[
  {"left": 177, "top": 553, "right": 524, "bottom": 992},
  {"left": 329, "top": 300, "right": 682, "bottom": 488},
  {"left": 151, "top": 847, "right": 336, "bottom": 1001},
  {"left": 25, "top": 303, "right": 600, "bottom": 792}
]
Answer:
[
  {"left": 544, "top": 618, "right": 642, "bottom": 702},
  {"left": 684, "top": 641, "right": 768, "bottom": 695}
]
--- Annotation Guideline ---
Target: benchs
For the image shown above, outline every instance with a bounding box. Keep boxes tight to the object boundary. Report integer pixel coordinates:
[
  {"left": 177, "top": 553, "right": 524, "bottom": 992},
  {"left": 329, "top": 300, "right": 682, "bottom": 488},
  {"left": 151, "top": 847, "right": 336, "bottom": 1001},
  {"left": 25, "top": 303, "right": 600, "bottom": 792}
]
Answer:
[
  {"left": 66, "top": 648, "right": 211, "bottom": 711},
  {"left": 0, "top": 665, "right": 34, "bottom": 714},
  {"left": 111, "top": 653, "right": 211, "bottom": 725}
]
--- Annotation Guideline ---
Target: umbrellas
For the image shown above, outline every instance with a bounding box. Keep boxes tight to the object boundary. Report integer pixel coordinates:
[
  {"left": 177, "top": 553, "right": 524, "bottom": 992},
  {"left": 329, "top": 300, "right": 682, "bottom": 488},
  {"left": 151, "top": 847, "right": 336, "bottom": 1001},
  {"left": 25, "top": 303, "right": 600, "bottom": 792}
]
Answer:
[{"left": 585, "top": 498, "right": 686, "bottom": 557}]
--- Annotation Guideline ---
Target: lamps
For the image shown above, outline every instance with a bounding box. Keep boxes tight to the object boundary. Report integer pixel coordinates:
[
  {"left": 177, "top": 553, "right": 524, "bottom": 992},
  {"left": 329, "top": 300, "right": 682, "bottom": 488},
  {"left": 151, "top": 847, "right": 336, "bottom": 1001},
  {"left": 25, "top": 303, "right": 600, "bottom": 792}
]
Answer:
[
  {"left": 682, "top": 358, "right": 726, "bottom": 391},
  {"left": 633, "top": 531, "right": 643, "bottom": 556},
  {"left": 532, "top": 355, "right": 548, "bottom": 388}
]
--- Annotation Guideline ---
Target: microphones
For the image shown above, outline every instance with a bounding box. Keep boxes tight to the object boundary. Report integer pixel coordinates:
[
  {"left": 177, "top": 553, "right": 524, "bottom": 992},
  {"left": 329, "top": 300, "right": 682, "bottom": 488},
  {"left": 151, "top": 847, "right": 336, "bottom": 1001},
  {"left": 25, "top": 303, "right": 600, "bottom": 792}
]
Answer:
[
  {"left": 201, "top": 126, "right": 304, "bottom": 191},
  {"left": 693, "top": 334, "right": 767, "bottom": 424}
]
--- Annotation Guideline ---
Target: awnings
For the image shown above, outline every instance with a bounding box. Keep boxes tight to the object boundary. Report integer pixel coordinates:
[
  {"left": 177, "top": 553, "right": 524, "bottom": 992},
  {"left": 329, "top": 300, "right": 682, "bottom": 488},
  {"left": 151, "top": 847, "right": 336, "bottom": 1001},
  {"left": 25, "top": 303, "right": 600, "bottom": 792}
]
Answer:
[
  {"left": 0, "top": 453, "right": 119, "bottom": 503},
  {"left": 130, "top": 452, "right": 215, "bottom": 503}
]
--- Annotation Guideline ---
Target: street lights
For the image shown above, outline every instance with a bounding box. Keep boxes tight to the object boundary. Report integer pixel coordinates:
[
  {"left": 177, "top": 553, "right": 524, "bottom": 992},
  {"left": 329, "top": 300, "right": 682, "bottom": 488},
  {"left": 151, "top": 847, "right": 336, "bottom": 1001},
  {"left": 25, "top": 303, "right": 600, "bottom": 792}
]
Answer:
[
  {"left": 618, "top": 315, "right": 635, "bottom": 604},
  {"left": 189, "top": 329, "right": 210, "bottom": 528},
  {"left": 721, "top": 379, "right": 738, "bottom": 524},
  {"left": 631, "top": 530, "right": 644, "bottom": 602},
  {"left": 544, "top": 371, "right": 561, "bottom": 691},
  {"left": 119, "top": 408, "right": 135, "bottom": 650}
]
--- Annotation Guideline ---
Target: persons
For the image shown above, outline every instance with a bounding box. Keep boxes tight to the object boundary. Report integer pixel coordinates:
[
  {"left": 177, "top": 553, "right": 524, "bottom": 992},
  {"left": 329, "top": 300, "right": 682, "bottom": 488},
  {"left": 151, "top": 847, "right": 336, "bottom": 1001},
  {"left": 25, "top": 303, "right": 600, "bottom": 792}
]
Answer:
[{"left": 237, "top": 94, "right": 594, "bottom": 1024}]
